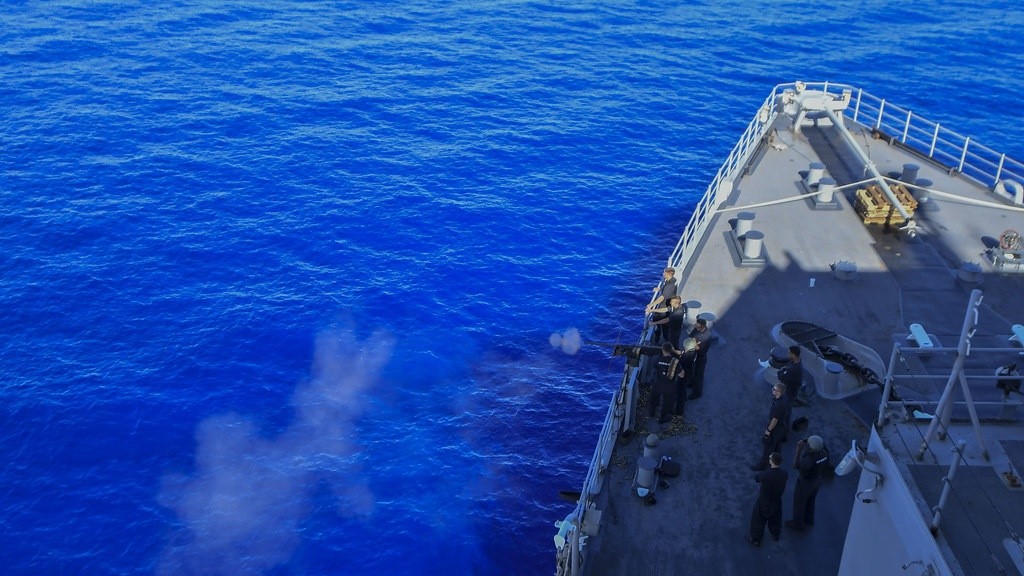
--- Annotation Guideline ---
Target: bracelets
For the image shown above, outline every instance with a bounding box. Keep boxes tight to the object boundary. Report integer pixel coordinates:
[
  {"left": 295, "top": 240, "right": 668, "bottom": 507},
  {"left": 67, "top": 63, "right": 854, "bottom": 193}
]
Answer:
[{"left": 766, "top": 426, "right": 772, "bottom": 432}]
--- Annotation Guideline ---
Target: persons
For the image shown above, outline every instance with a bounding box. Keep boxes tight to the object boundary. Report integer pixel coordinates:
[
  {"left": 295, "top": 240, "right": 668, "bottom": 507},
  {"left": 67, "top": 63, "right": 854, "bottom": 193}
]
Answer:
[
  {"left": 785, "top": 435, "right": 830, "bottom": 533},
  {"left": 743, "top": 451, "right": 790, "bottom": 548},
  {"left": 749, "top": 383, "right": 792, "bottom": 471},
  {"left": 642, "top": 268, "right": 714, "bottom": 424},
  {"left": 777, "top": 345, "right": 804, "bottom": 442}
]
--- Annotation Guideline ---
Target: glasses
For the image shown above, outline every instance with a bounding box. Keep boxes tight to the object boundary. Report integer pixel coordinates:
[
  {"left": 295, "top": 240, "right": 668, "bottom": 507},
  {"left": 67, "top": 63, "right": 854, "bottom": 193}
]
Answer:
[
  {"left": 694, "top": 324, "right": 703, "bottom": 329},
  {"left": 772, "top": 387, "right": 782, "bottom": 392},
  {"left": 768, "top": 457, "right": 772, "bottom": 459}
]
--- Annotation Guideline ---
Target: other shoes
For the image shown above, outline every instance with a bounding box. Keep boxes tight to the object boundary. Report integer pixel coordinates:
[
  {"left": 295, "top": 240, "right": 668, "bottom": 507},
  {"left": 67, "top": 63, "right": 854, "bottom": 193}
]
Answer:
[
  {"left": 804, "top": 514, "right": 814, "bottom": 526},
  {"left": 689, "top": 394, "right": 702, "bottom": 400},
  {"left": 656, "top": 409, "right": 673, "bottom": 423},
  {"left": 785, "top": 517, "right": 804, "bottom": 532},
  {"left": 751, "top": 464, "right": 768, "bottom": 471},
  {"left": 746, "top": 535, "right": 760, "bottom": 547},
  {"left": 672, "top": 410, "right": 684, "bottom": 418},
  {"left": 649, "top": 406, "right": 655, "bottom": 417},
  {"left": 772, "top": 533, "right": 778, "bottom": 540}
]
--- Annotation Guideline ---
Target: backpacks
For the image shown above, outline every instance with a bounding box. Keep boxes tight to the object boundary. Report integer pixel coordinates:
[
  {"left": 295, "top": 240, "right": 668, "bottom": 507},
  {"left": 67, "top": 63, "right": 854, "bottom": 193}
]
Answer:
[{"left": 813, "top": 450, "right": 834, "bottom": 482}]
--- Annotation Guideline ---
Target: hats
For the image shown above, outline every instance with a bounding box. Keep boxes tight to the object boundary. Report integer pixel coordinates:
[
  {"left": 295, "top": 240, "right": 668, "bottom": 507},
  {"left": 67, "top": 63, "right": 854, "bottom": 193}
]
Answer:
[{"left": 808, "top": 436, "right": 824, "bottom": 451}]
